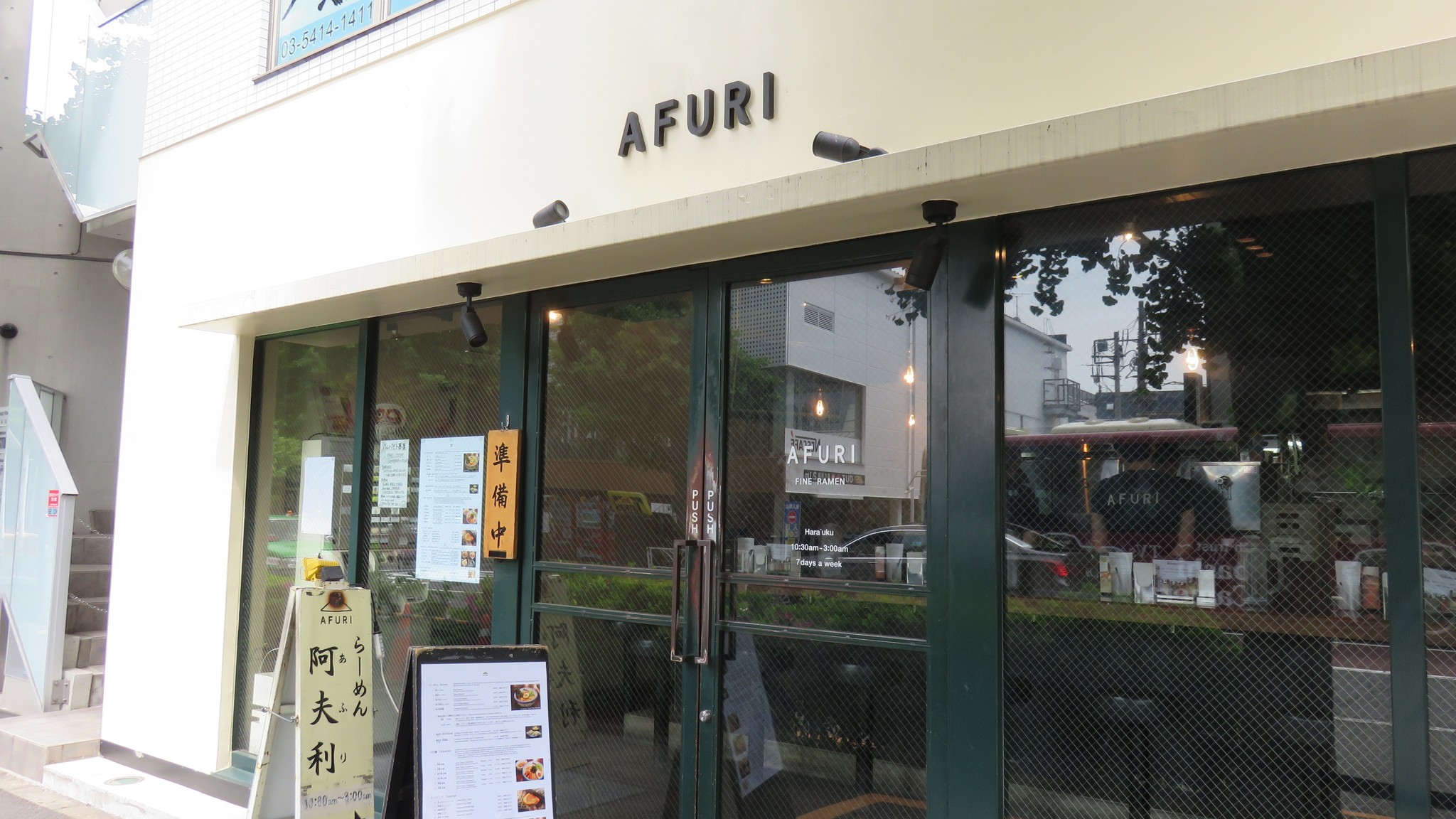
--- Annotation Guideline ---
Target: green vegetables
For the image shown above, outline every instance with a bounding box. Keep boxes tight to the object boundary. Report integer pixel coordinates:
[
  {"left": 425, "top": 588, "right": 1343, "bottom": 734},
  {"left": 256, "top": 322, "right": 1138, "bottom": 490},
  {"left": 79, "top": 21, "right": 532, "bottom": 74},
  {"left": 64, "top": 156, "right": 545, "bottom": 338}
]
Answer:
[
  {"left": 535, "top": 764, "right": 543, "bottom": 771},
  {"left": 529, "top": 691, "right": 534, "bottom": 697}
]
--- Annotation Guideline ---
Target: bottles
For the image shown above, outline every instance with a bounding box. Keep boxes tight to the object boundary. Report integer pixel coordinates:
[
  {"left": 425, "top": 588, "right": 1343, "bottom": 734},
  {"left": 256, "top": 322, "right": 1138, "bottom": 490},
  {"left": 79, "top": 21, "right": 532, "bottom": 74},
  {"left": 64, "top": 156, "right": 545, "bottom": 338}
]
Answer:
[
  {"left": 1423, "top": 590, "right": 1456, "bottom": 611},
  {"left": 768, "top": 557, "right": 790, "bottom": 571},
  {"left": 1161, "top": 576, "right": 1196, "bottom": 600}
]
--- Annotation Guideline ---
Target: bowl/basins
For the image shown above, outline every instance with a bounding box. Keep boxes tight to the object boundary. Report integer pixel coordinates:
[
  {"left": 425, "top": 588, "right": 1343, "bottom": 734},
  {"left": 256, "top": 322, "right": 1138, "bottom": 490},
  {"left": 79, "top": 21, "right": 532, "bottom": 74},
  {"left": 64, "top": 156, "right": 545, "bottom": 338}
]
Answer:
[
  {"left": 514, "top": 687, "right": 538, "bottom": 708},
  {"left": 466, "top": 510, "right": 477, "bottom": 524},
  {"left": 532, "top": 726, "right": 540, "bottom": 731},
  {"left": 522, "top": 761, "right": 544, "bottom": 780}
]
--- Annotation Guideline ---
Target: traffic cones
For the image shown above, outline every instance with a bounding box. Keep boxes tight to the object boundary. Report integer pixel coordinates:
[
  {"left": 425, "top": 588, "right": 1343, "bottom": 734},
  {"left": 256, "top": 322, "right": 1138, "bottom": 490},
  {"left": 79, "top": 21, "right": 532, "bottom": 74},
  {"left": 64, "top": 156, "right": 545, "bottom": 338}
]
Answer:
[
  {"left": 383, "top": 603, "right": 410, "bottom": 684},
  {"left": 475, "top": 613, "right": 491, "bottom": 645}
]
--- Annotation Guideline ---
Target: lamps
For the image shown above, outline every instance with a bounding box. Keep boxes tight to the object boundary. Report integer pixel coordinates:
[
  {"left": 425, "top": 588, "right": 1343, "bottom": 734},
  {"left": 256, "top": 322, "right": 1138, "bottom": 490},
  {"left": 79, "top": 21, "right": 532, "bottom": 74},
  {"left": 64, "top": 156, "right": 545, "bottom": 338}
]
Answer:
[
  {"left": 814, "top": 388, "right": 829, "bottom": 418},
  {"left": 532, "top": 200, "right": 569, "bottom": 229},
  {"left": 457, "top": 282, "right": 488, "bottom": 348},
  {"left": 905, "top": 200, "right": 959, "bottom": 291},
  {"left": 812, "top": 131, "right": 889, "bottom": 163}
]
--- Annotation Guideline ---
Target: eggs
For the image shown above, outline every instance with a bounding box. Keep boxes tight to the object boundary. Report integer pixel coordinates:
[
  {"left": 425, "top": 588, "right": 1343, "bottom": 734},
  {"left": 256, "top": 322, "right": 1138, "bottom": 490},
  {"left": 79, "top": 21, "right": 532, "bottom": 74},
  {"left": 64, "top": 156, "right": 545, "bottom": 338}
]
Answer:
[
  {"left": 536, "top": 770, "right": 542, "bottom": 779},
  {"left": 523, "top": 693, "right": 529, "bottom": 699}
]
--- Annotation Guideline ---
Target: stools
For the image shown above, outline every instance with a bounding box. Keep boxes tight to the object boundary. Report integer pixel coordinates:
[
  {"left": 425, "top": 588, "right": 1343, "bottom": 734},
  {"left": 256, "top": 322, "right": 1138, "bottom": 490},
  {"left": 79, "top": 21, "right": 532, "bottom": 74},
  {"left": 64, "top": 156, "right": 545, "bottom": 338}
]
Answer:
[
  {"left": 1105, "top": 693, "right": 1178, "bottom": 819},
  {"left": 837, "top": 658, "right": 900, "bottom": 796},
  {"left": 626, "top": 633, "right": 683, "bottom": 765}
]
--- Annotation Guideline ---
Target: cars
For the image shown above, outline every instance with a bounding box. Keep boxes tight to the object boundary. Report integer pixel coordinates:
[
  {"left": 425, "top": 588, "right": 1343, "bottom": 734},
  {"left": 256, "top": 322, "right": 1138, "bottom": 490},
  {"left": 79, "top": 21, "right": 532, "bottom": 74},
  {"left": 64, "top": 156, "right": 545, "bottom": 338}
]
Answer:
[
  {"left": 265, "top": 509, "right": 332, "bottom": 578},
  {"left": 1352, "top": 541, "right": 1456, "bottom": 600},
  {"left": 799, "top": 523, "right": 1126, "bottom": 594}
]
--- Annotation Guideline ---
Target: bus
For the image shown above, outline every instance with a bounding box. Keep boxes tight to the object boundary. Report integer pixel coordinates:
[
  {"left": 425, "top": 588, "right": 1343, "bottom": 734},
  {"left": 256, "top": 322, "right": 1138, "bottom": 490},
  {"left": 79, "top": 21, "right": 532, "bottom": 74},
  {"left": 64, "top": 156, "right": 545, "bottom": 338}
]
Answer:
[
  {"left": 1324, "top": 421, "right": 1456, "bottom": 574},
  {"left": 903, "top": 415, "right": 1245, "bottom": 607}
]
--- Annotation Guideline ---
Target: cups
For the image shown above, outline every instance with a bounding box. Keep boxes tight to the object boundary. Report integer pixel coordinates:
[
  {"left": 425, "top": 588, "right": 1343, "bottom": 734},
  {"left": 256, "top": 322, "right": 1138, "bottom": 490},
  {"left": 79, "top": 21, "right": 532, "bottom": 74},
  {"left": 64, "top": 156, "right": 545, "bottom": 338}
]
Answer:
[
  {"left": 469, "top": 552, "right": 475, "bottom": 559},
  {"left": 463, "top": 508, "right": 469, "bottom": 516},
  {"left": 1235, "top": 538, "right": 1283, "bottom": 605},
  {"left": 737, "top": 549, "right": 753, "bottom": 573},
  {"left": 465, "top": 456, "right": 478, "bottom": 470},
  {"left": 1334, "top": 561, "right": 1361, "bottom": 611},
  {"left": 469, "top": 560, "right": 475, "bottom": 566},
  {"left": 462, "top": 551, "right": 468, "bottom": 558},
  {"left": 516, "top": 760, "right": 528, "bottom": 771},
  {"left": 886, "top": 543, "right": 903, "bottom": 582}
]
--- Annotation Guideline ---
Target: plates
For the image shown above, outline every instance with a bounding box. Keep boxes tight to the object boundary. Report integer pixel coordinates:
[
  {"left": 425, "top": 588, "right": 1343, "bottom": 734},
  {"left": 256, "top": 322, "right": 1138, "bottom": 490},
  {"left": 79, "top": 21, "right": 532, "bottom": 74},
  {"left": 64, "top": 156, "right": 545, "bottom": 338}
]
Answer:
[{"left": 526, "top": 730, "right": 541, "bottom": 737}]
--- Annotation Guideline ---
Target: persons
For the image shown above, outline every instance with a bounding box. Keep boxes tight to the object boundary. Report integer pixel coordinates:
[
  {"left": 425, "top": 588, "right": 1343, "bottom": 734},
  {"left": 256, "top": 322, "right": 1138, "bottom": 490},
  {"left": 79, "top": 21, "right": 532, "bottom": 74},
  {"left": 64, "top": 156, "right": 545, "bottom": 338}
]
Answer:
[
  {"left": 1003, "top": 447, "right": 1040, "bottom": 550},
  {"left": 1088, "top": 435, "right": 1199, "bottom": 561}
]
